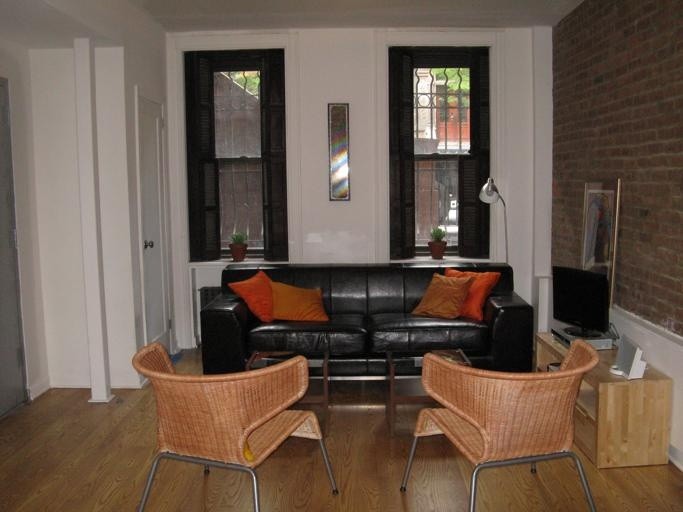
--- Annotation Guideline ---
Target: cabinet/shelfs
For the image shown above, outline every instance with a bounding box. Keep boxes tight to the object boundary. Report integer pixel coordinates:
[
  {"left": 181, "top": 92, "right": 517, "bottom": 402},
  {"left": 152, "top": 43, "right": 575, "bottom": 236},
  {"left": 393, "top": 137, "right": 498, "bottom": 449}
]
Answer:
[{"left": 532, "top": 330, "right": 674, "bottom": 471}]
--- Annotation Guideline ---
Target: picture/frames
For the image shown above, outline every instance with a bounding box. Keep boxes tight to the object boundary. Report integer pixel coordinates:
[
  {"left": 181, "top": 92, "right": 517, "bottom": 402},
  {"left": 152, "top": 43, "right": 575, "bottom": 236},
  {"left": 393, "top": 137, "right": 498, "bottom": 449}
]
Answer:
[
  {"left": 327, "top": 102, "right": 351, "bottom": 201},
  {"left": 579, "top": 177, "right": 621, "bottom": 308}
]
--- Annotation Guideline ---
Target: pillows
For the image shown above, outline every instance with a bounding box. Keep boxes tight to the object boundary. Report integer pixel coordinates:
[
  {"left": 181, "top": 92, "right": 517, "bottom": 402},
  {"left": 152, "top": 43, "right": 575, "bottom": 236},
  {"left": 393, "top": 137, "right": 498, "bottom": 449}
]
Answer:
[
  {"left": 408, "top": 269, "right": 501, "bottom": 321},
  {"left": 228, "top": 271, "right": 330, "bottom": 323}
]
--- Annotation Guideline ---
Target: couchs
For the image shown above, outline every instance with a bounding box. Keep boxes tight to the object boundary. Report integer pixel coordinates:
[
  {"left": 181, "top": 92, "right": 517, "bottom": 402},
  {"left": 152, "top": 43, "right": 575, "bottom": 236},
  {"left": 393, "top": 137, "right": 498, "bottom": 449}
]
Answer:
[{"left": 198, "top": 262, "right": 535, "bottom": 374}]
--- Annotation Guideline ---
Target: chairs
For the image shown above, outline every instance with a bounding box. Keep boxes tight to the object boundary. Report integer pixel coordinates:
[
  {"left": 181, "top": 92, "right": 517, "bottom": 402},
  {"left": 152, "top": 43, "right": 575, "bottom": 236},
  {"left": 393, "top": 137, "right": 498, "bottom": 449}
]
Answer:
[
  {"left": 399, "top": 340, "right": 600, "bottom": 511},
  {"left": 132, "top": 343, "right": 339, "bottom": 512}
]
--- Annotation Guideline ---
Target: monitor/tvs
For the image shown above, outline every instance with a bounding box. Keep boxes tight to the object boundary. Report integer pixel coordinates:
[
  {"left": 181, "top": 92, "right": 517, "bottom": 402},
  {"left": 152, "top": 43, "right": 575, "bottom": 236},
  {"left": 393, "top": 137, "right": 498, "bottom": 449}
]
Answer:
[{"left": 552, "top": 265, "right": 610, "bottom": 339}]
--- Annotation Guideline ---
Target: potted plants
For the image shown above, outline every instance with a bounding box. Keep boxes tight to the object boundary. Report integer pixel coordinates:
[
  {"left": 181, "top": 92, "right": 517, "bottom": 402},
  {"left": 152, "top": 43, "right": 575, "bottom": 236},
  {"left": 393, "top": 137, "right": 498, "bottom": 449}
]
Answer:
[
  {"left": 229, "top": 232, "right": 248, "bottom": 261},
  {"left": 428, "top": 228, "right": 448, "bottom": 259}
]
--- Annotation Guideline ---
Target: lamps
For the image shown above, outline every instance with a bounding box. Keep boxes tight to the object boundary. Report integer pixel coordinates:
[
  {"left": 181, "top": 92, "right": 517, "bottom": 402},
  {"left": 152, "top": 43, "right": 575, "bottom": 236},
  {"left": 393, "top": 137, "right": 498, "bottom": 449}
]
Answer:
[{"left": 479, "top": 177, "right": 509, "bottom": 264}]
